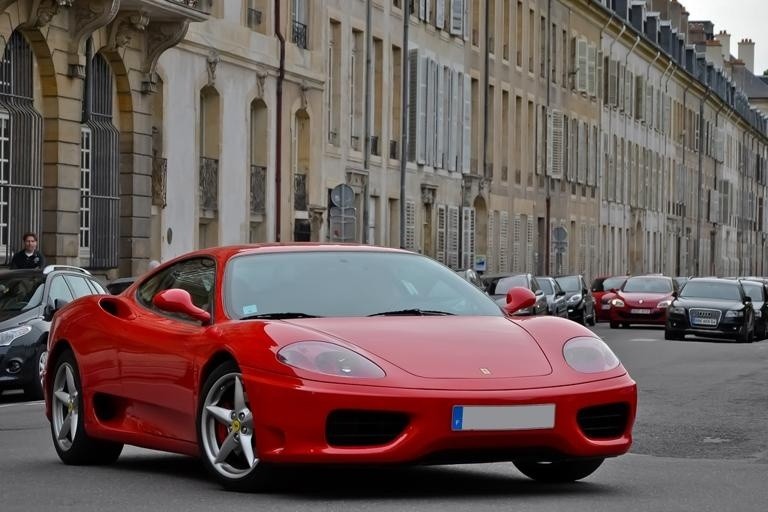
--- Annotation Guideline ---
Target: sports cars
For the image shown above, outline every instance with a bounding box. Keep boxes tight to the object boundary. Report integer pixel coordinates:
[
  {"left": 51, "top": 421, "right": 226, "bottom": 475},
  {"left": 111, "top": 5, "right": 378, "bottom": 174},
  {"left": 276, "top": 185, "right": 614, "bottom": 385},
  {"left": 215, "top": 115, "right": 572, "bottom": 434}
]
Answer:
[{"left": 41, "top": 239, "right": 645, "bottom": 495}]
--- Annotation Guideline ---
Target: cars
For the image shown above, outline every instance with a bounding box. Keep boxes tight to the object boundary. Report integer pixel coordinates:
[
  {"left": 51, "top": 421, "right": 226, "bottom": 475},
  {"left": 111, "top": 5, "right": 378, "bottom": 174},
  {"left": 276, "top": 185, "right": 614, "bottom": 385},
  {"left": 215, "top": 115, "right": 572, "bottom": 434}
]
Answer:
[
  {"left": 106, "top": 277, "right": 142, "bottom": 296},
  {"left": 0, "top": 262, "right": 112, "bottom": 402}
]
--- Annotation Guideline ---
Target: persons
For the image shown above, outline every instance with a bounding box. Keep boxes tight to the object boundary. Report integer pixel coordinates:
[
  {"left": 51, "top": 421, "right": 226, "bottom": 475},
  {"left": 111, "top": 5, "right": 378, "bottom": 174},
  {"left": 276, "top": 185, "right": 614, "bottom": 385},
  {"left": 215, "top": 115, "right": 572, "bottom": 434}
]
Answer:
[{"left": 11, "top": 233, "right": 46, "bottom": 270}]
